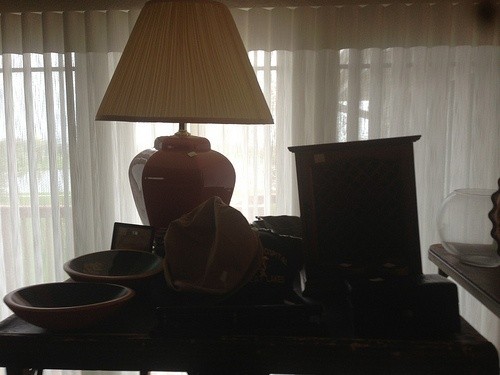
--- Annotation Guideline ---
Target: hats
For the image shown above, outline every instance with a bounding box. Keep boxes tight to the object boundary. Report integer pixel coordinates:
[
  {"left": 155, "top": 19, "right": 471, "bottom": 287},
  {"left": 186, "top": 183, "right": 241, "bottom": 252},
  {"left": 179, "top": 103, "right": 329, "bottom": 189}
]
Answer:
[{"left": 165, "top": 194, "right": 262, "bottom": 295}]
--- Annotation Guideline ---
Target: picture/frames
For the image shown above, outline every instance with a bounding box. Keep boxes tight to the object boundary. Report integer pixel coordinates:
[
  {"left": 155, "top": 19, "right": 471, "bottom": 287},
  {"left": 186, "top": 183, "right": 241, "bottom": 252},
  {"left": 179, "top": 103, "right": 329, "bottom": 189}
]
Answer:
[{"left": 110, "top": 221, "right": 154, "bottom": 253}]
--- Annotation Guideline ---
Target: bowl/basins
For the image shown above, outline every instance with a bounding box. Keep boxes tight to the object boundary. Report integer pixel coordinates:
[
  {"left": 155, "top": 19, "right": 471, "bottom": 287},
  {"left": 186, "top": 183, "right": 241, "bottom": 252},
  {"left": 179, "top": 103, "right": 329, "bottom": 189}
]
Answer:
[
  {"left": 63, "top": 249, "right": 164, "bottom": 289},
  {"left": 2, "top": 282, "right": 134, "bottom": 330}
]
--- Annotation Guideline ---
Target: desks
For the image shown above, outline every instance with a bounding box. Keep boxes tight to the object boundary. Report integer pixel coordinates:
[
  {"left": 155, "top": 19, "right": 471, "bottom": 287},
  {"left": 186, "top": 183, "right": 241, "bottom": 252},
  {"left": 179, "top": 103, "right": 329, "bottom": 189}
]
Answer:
[
  {"left": 0, "top": 278, "right": 500, "bottom": 375},
  {"left": 429, "top": 244, "right": 500, "bottom": 319}
]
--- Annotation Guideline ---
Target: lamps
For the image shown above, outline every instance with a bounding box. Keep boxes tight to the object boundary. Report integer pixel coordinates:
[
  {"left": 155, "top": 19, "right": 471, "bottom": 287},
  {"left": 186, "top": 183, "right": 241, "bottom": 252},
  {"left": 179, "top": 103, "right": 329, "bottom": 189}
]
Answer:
[{"left": 95, "top": 0, "right": 275, "bottom": 243}]
